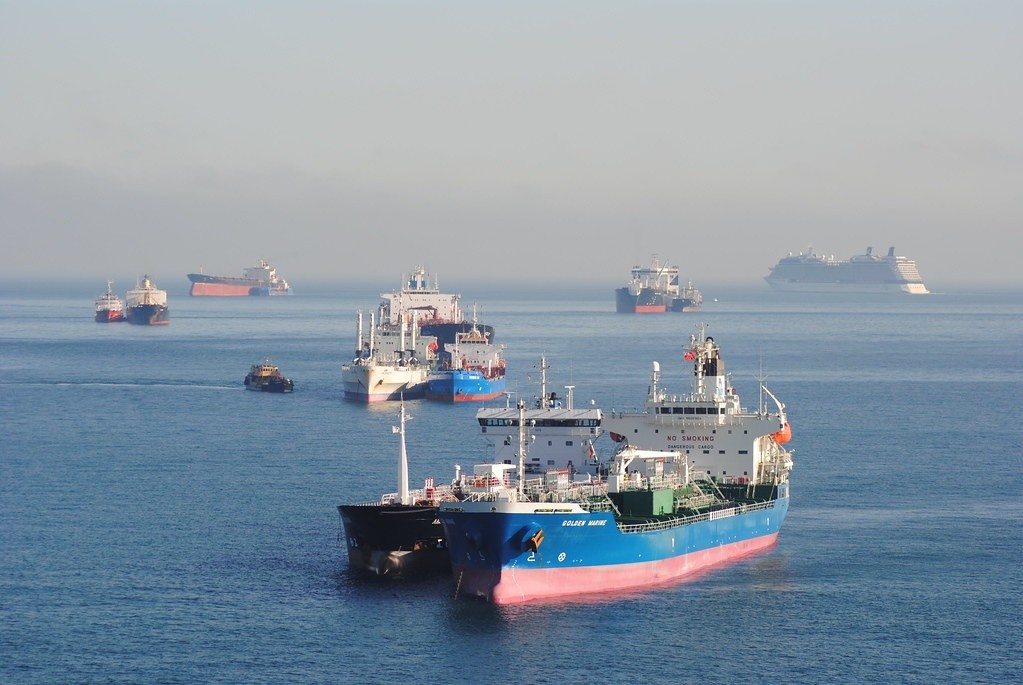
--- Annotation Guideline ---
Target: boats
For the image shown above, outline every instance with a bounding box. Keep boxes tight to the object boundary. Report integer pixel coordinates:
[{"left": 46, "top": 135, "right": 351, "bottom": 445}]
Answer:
[
  {"left": 339, "top": 263, "right": 465, "bottom": 405},
  {"left": 242, "top": 358, "right": 296, "bottom": 395},
  {"left": 761, "top": 244, "right": 931, "bottom": 296},
  {"left": 123, "top": 273, "right": 171, "bottom": 325},
  {"left": 613, "top": 253, "right": 704, "bottom": 314},
  {"left": 333, "top": 356, "right": 604, "bottom": 579},
  {"left": 184, "top": 259, "right": 293, "bottom": 298},
  {"left": 437, "top": 321, "right": 797, "bottom": 609},
  {"left": 93, "top": 279, "right": 124, "bottom": 324},
  {"left": 425, "top": 294, "right": 508, "bottom": 404}
]
